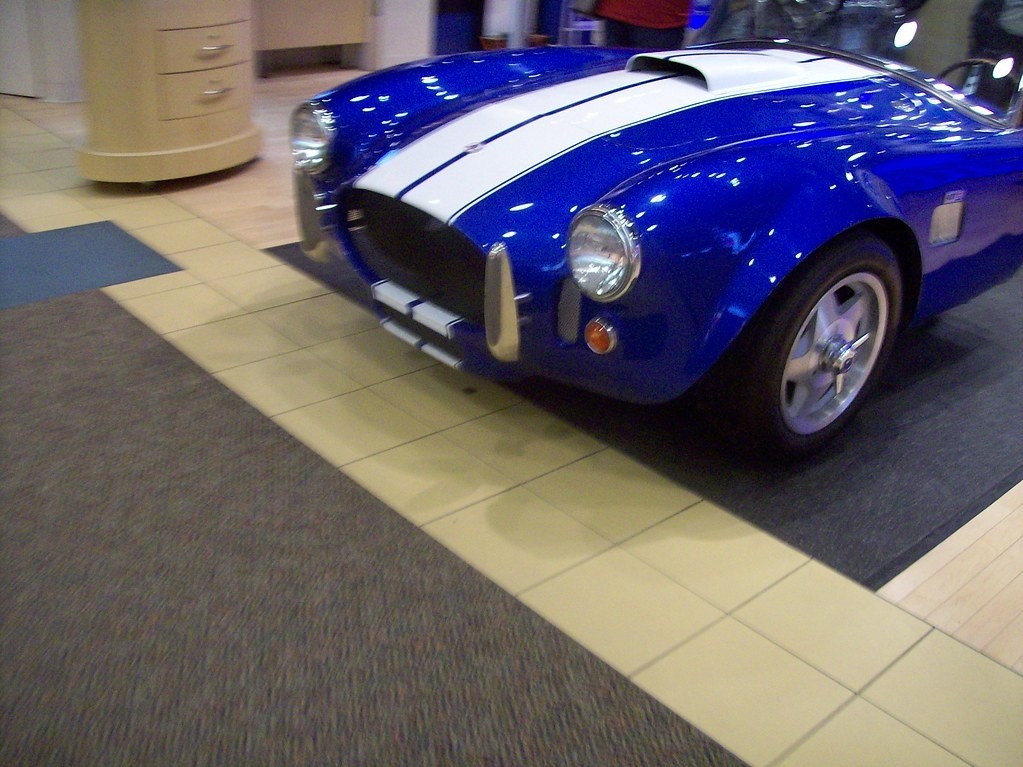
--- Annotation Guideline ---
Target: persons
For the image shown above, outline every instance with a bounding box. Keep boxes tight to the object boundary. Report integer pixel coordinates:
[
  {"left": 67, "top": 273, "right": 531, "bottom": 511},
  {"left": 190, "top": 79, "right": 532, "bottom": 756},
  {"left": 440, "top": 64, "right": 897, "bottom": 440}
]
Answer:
[{"left": 594, "top": 0, "right": 691, "bottom": 47}]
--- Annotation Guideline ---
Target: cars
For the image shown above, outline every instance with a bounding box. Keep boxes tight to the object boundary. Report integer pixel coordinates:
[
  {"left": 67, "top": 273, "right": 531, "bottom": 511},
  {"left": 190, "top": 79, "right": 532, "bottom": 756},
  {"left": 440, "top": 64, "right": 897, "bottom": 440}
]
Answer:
[{"left": 289, "top": 0, "right": 1023, "bottom": 456}]
